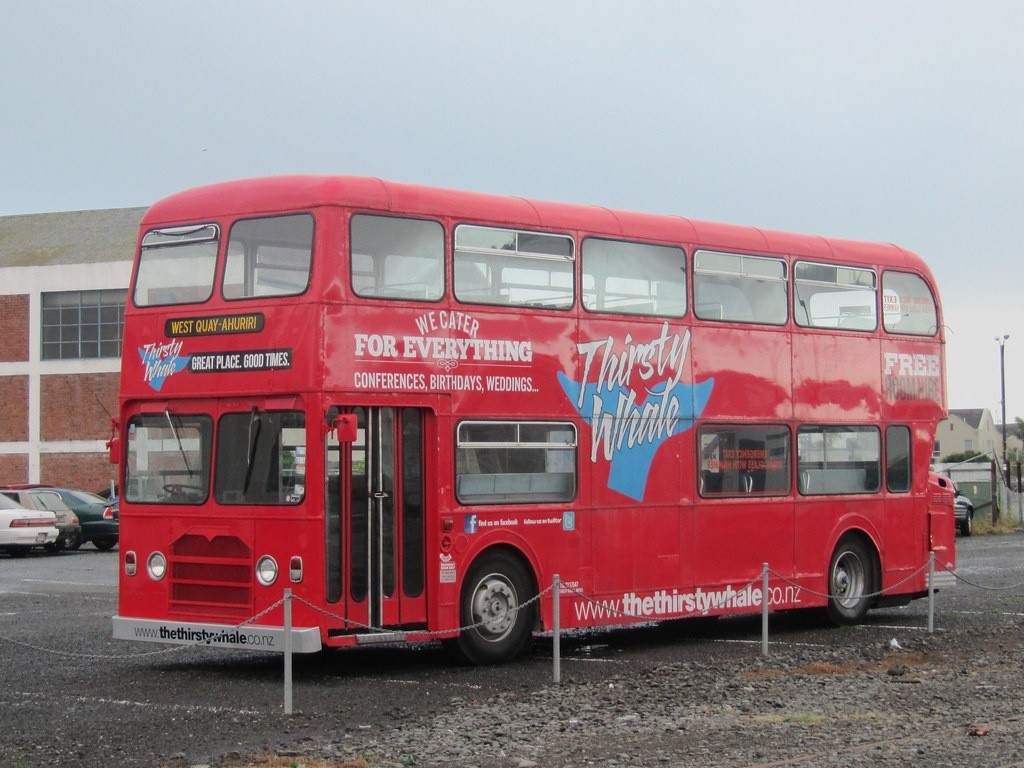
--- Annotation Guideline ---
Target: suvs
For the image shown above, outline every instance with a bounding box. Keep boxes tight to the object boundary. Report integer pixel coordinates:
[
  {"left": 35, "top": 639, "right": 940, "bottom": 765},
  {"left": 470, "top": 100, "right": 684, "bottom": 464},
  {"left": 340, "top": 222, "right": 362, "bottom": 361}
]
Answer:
[{"left": 2, "top": 490, "right": 82, "bottom": 552}]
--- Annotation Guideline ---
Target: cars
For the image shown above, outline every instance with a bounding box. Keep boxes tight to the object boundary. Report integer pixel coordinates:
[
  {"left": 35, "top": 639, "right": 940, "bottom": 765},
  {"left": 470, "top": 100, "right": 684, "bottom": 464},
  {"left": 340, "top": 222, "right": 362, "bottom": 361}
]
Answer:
[
  {"left": 31, "top": 487, "right": 120, "bottom": 550},
  {"left": 1, "top": 491, "right": 60, "bottom": 557},
  {"left": 954, "top": 485, "right": 974, "bottom": 536}
]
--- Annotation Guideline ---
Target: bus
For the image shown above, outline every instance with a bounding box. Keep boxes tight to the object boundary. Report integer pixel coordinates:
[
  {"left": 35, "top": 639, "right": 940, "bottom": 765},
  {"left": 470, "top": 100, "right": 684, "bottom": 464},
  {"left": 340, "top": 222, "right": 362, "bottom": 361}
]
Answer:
[{"left": 104, "top": 173, "right": 957, "bottom": 664}]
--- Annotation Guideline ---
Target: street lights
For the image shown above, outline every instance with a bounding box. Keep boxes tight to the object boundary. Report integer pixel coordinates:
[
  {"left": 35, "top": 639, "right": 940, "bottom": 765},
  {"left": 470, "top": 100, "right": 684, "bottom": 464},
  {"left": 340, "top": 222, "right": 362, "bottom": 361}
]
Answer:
[{"left": 995, "top": 335, "right": 1011, "bottom": 468}]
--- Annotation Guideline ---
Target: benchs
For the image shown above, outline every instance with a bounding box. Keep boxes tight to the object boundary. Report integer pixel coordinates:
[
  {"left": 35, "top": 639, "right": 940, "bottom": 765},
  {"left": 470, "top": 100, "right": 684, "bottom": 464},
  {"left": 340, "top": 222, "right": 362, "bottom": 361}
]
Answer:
[
  {"left": 359, "top": 282, "right": 929, "bottom": 335},
  {"left": 696, "top": 461, "right": 868, "bottom": 495}
]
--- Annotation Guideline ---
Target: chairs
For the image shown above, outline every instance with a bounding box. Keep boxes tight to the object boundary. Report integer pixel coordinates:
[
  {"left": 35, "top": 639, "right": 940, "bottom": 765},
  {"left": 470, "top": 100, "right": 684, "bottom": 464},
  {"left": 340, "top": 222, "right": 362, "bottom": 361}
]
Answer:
[
  {"left": 222, "top": 490, "right": 261, "bottom": 505},
  {"left": 456, "top": 466, "right": 574, "bottom": 508}
]
